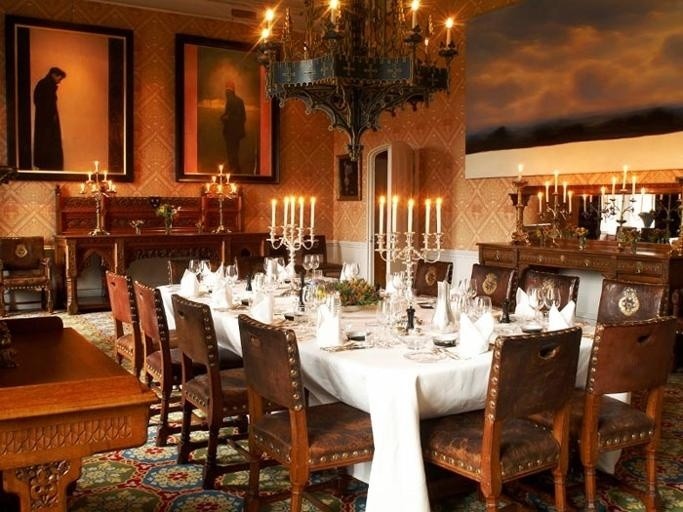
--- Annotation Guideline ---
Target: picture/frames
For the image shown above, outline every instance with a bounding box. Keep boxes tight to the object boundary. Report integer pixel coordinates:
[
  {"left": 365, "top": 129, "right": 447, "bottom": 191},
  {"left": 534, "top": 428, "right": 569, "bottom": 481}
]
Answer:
[
  {"left": 4, "top": 13, "right": 134, "bottom": 184},
  {"left": 333, "top": 152, "right": 364, "bottom": 201},
  {"left": 173, "top": 29, "right": 283, "bottom": 184}
]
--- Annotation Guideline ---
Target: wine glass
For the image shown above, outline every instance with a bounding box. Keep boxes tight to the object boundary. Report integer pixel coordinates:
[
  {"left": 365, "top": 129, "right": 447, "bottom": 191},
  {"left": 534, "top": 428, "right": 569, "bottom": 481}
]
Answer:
[{"left": 188, "top": 254, "right": 561, "bottom": 341}]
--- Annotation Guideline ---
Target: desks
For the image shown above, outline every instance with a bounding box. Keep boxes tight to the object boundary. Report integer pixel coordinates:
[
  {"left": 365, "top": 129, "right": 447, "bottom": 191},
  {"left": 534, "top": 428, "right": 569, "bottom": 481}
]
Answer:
[{"left": 0, "top": 316, "right": 160, "bottom": 512}]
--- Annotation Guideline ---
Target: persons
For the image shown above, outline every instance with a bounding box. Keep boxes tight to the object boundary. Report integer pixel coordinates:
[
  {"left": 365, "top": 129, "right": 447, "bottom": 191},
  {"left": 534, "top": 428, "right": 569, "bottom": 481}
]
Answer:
[
  {"left": 220, "top": 78, "right": 246, "bottom": 174},
  {"left": 32, "top": 67, "right": 66, "bottom": 171}
]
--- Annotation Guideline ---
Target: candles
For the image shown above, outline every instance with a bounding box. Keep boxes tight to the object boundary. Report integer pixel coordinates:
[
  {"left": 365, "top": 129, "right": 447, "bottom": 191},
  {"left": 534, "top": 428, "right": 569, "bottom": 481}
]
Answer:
[
  {"left": 518, "top": 163, "right": 646, "bottom": 215},
  {"left": 81, "top": 160, "right": 116, "bottom": 193},
  {"left": 204, "top": 165, "right": 238, "bottom": 195},
  {"left": 270, "top": 195, "right": 315, "bottom": 229},
  {"left": 380, "top": 194, "right": 453, "bottom": 238}
]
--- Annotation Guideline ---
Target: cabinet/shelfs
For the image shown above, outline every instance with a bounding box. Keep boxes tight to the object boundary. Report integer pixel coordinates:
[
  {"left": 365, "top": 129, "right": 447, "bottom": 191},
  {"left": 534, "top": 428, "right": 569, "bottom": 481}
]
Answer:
[
  {"left": 53, "top": 185, "right": 272, "bottom": 317},
  {"left": 476, "top": 184, "right": 682, "bottom": 310}
]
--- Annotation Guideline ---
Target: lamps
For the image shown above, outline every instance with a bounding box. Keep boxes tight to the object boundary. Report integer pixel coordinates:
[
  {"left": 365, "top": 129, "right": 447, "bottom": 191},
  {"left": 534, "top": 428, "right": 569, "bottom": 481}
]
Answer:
[{"left": 259, "top": 0, "right": 457, "bottom": 163}]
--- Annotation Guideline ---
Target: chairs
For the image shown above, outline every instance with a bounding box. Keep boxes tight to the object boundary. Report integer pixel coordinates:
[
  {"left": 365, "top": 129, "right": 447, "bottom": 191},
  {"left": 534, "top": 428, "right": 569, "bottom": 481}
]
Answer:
[
  {"left": 413, "top": 260, "right": 668, "bottom": 324},
  {"left": 167, "top": 255, "right": 212, "bottom": 287},
  {"left": 422, "top": 329, "right": 582, "bottom": 511},
  {"left": 0, "top": 237, "right": 57, "bottom": 315},
  {"left": 530, "top": 313, "right": 678, "bottom": 512},
  {"left": 273, "top": 233, "right": 349, "bottom": 274},
  {"left": 233, "top": 256, "right": 269, "bottom": 279}
]
